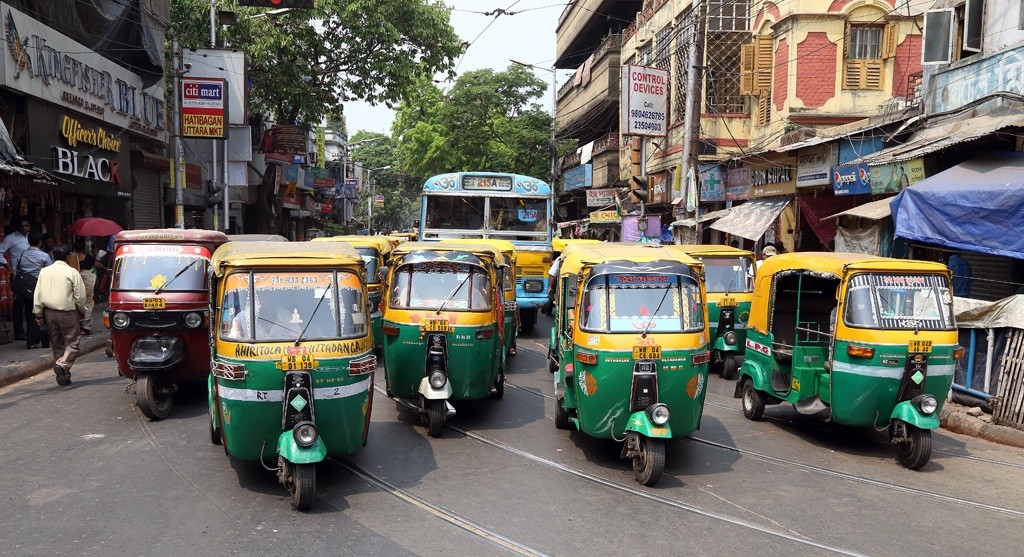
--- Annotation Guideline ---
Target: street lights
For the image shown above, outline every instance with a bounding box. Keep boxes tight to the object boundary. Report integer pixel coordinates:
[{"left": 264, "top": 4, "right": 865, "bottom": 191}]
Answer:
[
  {"left": 338, "top": 137, "right": 380, "bottom": 225},
  {"left": 509, "top": 59, "right": 558, "bottom": 235},
  {"left": 361, "top": 166, "right": 391, "bottom": 237},
  {"left": 223, "top": 7, "right": 296, "bottom": 236}
]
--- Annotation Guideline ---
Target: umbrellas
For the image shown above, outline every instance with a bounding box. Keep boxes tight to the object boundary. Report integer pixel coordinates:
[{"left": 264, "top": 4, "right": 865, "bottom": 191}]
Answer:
[{"left": 68, "top": 217, "right": 123, "bottom": 261}]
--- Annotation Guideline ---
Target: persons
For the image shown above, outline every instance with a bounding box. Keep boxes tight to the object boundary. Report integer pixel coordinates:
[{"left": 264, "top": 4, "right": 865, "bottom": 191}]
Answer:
[
  {"left": 41, "top": 233, "right": 60, "bottom": 263},
  {"left": 72, "top": 240, "right": 113, "bottom": 334},
  {"left": 16, "top": 231, "right": 53, "bottom": 349},
  {"left": 749, "top": 241, "right": 785, "bottom": 288},
  {"left": 732, "top": 239, "right": 739, "bottom": 247},
  {"left": 0, "top": 218, "right": 32, "bottom": 340},
  {"left": 829, "top": 304, "right": 839, "bottom": 359},
  {"left": 32, "top": 245, "right": 88, "bottom": 387},
  {"left": 89, "top": 235, "right": 117, "bottom": 303},
  {"left": 227, "top": 290, "right": 305, "bottom": 339}
]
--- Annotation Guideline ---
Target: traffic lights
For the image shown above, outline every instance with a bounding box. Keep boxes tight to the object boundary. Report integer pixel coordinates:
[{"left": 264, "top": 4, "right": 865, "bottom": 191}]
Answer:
[
  {"left": 632, "top": 174, "right": 649, "bottom": 204},
  {"left": 233, "top": 0, "right": 315, "bottom": 9}
]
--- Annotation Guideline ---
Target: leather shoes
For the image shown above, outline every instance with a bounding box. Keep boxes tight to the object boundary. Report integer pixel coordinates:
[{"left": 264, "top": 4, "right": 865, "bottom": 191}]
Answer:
[{"left": 53, "top": 365, "right": 68, "bottom": 386}]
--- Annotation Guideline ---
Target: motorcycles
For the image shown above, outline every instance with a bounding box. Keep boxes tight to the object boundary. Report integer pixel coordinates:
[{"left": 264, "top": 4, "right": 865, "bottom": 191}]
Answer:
[
  {"left": 218, "top": 231, "right": 606, "bottom": 374},
  {"left": 554, "top": 241, "right": 710, "bottom": 486},
  {"left": 100, "top": 227, "right": 229, "bottom": 425},
  {"left": 206, "top": 241, "right": 378, "bottom": 512},
  {"left": 378, "top": 243, "right": 507, "bottom": 440},
  {"left": 732, "top": 250, "right": 966, "bottom": 471},
  {"left": 660, "top": 244, "right": 761, "bottom": 380}
]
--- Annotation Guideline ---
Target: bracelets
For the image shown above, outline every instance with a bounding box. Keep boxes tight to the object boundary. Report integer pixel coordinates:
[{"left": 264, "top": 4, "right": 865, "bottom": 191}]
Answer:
[{"left": 36, "top": 316, "right": 42, "bottom": 320}]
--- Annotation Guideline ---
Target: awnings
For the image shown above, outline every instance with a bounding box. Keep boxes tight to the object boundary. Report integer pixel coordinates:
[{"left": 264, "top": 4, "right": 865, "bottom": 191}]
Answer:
[
  {"left": 667, "top": 142, "right": 803, "bottom": 242},
  {"left": 776, "top": 105, "right": 1023, "bottom": 196},
  {"left": 889, "top": 151, "right": 1024, "bottom": 260}
]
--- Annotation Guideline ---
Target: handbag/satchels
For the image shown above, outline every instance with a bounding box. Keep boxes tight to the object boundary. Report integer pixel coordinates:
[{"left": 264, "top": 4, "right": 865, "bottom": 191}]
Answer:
[{"left": 10, "top": 249, "right": 38, "bottom": 301}]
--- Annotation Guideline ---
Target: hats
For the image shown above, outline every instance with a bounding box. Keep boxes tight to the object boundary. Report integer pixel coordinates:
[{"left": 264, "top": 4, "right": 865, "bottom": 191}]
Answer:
[{"left": 763, "top": 245, "right": 779, "bottom": 257}]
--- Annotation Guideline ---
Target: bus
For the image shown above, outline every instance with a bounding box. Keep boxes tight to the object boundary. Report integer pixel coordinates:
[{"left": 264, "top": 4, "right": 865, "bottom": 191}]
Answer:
[{"left": 413, "top": 170, "right": 558, "bottom": 338}]
